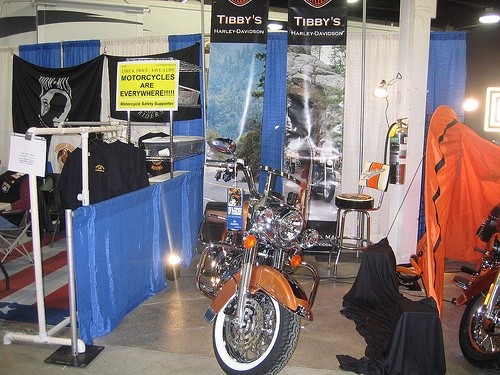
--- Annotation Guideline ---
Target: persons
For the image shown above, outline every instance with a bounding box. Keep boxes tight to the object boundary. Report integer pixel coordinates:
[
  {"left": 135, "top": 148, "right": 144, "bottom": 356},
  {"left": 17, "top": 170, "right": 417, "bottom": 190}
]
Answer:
[{"left": 0, "top": 170, "right": 30, "bottom": 230}]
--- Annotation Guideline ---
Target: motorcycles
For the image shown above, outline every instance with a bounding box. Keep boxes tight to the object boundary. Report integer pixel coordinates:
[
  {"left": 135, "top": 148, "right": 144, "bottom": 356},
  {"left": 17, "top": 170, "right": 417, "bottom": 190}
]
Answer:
[
  {"left": 284, "top": 144, "right": 335, "bottom": 202},
  {"left": 195, "top": 138, "right": 320, "bottom": 375},
  {"left": 214, "top": 161, "right": 238, "bottom": 181},
  {"left": 452, "top": 203, "right": 500, "bottom": 367}
]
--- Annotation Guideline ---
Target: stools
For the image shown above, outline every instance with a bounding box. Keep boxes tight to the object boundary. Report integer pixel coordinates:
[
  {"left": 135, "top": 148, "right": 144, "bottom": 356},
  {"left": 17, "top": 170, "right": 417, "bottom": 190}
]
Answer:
[{"left": 327, "top": 162, "right": 392, "bottom": 279}]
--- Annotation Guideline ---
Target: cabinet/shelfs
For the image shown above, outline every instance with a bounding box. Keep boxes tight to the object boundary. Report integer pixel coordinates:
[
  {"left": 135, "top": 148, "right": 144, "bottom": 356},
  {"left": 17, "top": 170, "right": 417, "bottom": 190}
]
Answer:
[{"left": 125, "top": 57, "right": 206, "bottom": 179}]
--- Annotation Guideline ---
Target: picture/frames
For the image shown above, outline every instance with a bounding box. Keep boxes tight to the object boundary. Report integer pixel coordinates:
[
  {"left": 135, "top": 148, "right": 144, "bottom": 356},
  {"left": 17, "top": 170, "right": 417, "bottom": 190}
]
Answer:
[{"left": 484, "top": 86, "right": 500, "bottom": 132}]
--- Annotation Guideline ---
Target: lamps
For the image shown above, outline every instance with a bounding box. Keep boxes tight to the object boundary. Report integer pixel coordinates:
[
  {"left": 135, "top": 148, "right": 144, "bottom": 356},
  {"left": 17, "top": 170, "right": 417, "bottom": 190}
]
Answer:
[
  {"left": 478, "top": 7, "right": 500, "bottom": 24},
  {"left": 373, "top": 79, "right": 389, "bottom": 99}
]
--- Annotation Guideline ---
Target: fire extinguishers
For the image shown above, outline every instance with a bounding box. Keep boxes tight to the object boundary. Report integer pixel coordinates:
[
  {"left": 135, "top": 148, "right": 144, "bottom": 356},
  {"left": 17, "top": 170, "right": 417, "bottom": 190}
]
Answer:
[{"left": 384, "top": 117, "right": 408, "bottom": 185}]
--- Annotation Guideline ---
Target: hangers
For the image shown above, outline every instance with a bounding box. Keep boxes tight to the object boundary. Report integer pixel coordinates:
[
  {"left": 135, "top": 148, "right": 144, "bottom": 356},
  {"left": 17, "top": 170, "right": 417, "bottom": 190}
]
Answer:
[{"left": 77, "top": 125, "right": 130, "bottom": 147}]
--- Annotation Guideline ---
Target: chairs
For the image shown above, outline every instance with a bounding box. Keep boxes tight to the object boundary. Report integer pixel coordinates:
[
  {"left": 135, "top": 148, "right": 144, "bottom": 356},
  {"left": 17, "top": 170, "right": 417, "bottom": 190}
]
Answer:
[{"left": 0, "top": 209, "right": 33, "bottom": 265}]
